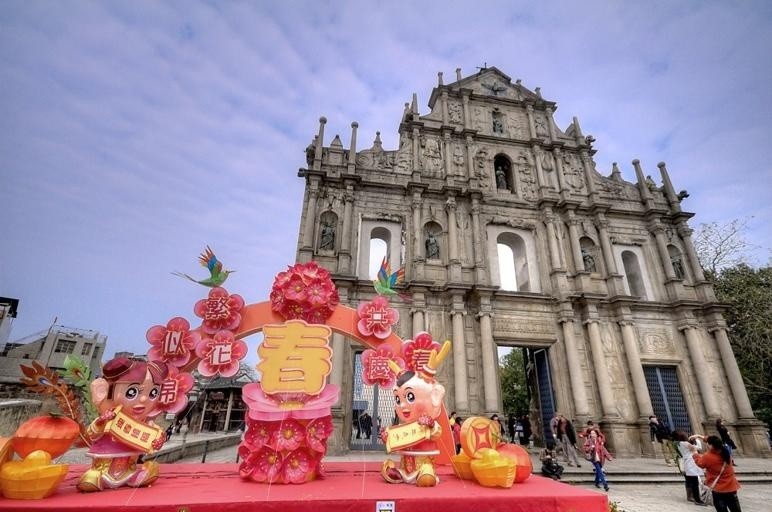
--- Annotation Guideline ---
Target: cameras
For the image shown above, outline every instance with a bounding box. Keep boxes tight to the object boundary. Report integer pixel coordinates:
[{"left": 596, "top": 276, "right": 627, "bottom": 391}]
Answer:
[{"left": 688, "top": 438, "right": 696, "bottom": 445}]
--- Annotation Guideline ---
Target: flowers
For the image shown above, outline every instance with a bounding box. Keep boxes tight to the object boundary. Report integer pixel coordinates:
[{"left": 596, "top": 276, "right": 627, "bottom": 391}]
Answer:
[
  {"left": 233, "top": 382, "right": 341, "bottom": 486},
  {"left": 270, "top": 261, "right": 341, "bottom": 323},
  {"left": 135, "top": 286, "right": 249, "bottom": 420},
  {"left": 359, "top": 297, "right": 443, "bottom": 391}
]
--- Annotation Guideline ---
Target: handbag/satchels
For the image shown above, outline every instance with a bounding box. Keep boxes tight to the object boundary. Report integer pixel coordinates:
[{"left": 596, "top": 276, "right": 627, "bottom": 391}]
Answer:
[
  {"left": 583, "top": 444, "right": 593, "bottom": 459},
  {"left": 698, "top": 483, "right": 714, "bottom": 506}
]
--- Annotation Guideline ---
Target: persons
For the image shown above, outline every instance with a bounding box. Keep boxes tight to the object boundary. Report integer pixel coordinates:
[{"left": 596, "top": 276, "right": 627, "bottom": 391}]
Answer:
[
  {"left": 353, "top": 415, "right": 361, "bottom": 439},
  {"left": 359, "top": 412, "right": 374, "bottom": 439},
  {"left": 448, "top": 412, "right": 612, "bottom": 491},
  {"left": 649, "top": 414, "right": 741, "bottom": 512}
]
